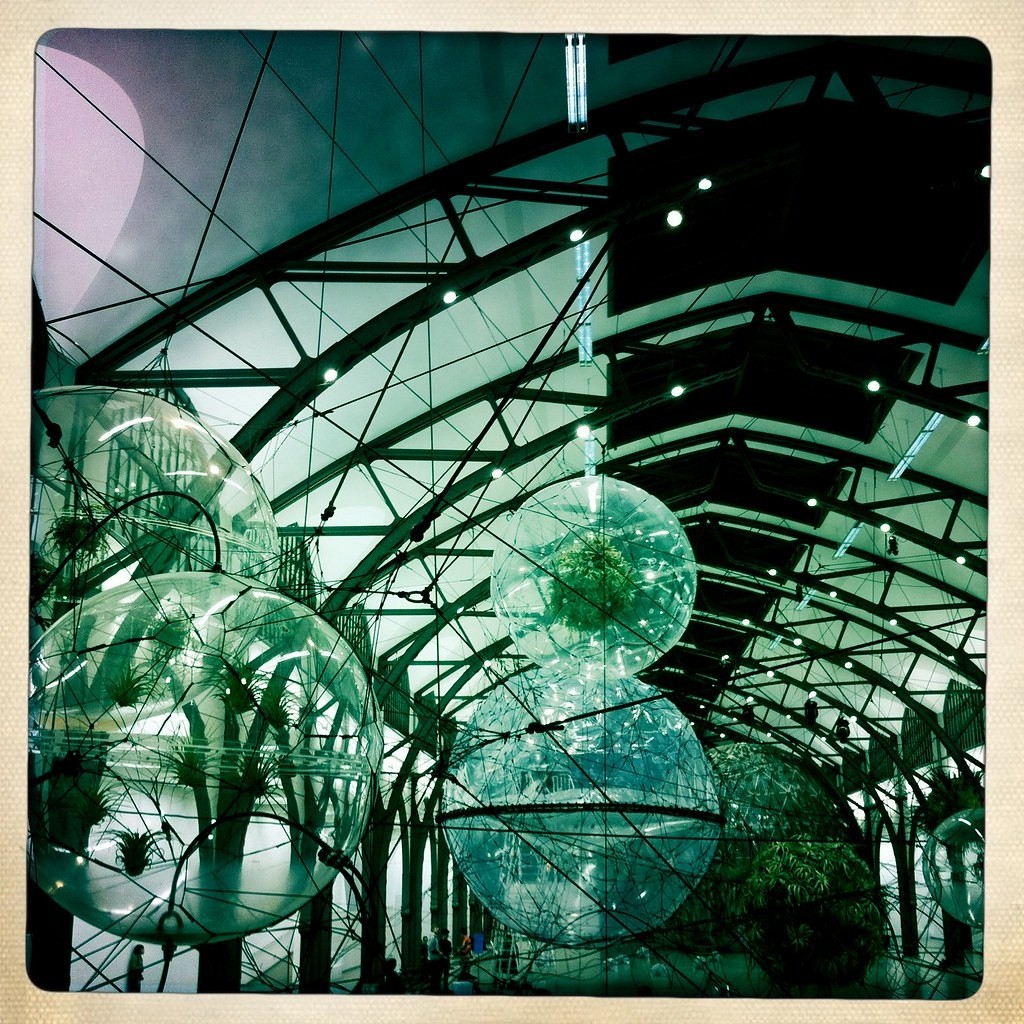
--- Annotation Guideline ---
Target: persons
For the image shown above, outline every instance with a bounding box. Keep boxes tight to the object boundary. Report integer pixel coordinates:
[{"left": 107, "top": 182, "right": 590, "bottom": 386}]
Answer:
[
  {"left": 382, "top": 958, "right": 405, "bottom": 994},
  {"left": 421, "top": 937, "right": 429, "bottom": 960},
  {"left": 126, "top": 945, "right": 144, "bottom": 992},
  {"left": 458, "top": 928, "right": 476, "bottom": 980},
  {"left": 426, "top": 924, "right": 448, "bottom": 995},
  {"left": 437, "top": 928, "right": 454, "bottom": 994}
]
